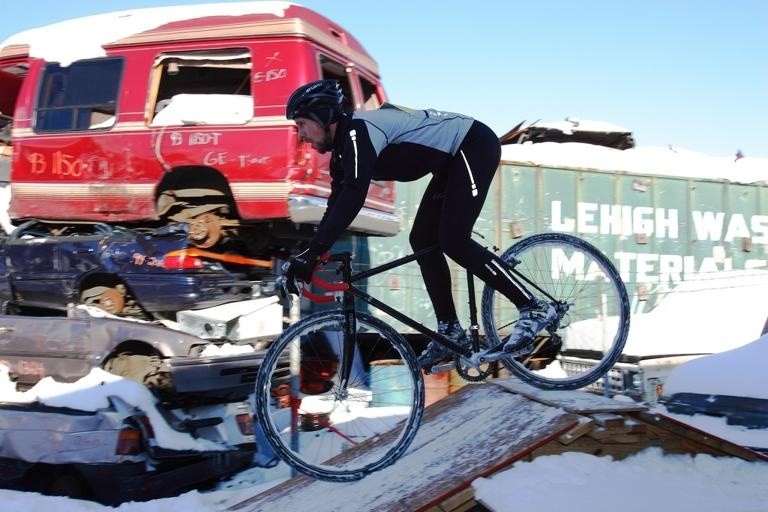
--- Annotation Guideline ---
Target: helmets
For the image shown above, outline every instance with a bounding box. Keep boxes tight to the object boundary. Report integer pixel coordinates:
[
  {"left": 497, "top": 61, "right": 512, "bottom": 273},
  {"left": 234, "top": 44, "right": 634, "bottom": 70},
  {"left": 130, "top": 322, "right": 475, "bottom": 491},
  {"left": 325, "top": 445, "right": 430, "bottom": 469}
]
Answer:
[{"left": 285, "top": 78, "right": 345, "bottom": 119}]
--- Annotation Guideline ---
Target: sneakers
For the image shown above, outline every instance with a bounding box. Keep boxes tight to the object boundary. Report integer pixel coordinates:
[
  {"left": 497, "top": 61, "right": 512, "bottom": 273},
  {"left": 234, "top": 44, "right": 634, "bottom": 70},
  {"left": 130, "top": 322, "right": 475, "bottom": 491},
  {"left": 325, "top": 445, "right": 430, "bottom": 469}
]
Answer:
[
  {"left": 503, "top": 297, "right": 560, "bottom": 355},
  {"left": 415, "top": 318, "right": 470, "bottom": 366}
]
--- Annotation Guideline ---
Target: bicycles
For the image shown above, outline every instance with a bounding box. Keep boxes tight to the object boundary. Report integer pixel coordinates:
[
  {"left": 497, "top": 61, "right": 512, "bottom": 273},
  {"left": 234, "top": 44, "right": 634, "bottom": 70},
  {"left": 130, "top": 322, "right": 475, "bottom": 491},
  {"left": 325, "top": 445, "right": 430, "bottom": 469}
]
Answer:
[{"left": 250, "top": 231, "right": 630, "bottom": 482}]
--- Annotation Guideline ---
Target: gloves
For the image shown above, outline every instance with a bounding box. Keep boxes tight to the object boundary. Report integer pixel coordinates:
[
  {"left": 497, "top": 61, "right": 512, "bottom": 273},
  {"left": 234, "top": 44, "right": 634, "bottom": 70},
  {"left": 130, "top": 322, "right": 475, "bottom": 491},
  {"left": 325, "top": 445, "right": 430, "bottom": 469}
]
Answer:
[{"left": 280, "top": 247, "right": 320, "bottom": 300}]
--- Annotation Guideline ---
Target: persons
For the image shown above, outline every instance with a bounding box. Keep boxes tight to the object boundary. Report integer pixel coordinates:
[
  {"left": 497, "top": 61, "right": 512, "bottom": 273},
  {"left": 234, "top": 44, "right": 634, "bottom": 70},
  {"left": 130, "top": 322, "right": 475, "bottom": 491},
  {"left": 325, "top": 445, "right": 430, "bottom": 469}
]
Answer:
[{"left": 281, "top": 78, "right": 561, "bottom": 373}]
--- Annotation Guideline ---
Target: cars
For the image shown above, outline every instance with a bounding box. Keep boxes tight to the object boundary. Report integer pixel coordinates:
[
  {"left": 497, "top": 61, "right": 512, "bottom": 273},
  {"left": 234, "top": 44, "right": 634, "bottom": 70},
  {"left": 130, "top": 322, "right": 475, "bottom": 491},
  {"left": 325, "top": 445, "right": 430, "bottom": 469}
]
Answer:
[{"left": 550, "top": 269, "right": 767, "bottom": 453}]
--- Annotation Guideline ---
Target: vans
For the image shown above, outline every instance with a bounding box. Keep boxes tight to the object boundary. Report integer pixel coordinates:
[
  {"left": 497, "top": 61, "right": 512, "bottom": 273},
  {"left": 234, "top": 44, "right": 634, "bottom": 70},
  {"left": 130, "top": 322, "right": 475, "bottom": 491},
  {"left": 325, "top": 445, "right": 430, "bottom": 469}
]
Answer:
[{"left": 0, "top": 2, "right": 399, "bottom": 241}]
